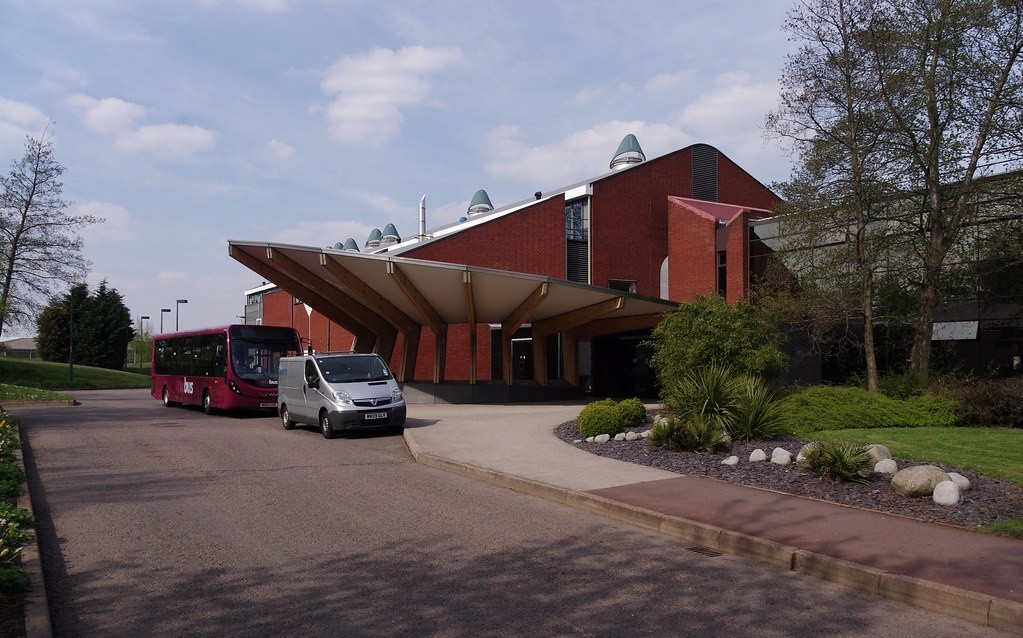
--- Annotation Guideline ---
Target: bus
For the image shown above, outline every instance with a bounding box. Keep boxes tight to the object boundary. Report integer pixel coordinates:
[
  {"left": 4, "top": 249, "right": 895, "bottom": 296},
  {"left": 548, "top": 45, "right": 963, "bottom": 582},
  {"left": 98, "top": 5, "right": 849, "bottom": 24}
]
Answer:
[{"left": 151, "top": 324, "right": 313, "bottom": 415}]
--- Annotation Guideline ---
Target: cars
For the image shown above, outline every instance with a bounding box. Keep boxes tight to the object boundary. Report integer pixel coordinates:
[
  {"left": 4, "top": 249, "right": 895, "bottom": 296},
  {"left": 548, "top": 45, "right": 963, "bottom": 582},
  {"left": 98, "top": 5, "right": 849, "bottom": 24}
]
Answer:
[{"left": 277, "top": 352, "right": 406, "bottom": 440}]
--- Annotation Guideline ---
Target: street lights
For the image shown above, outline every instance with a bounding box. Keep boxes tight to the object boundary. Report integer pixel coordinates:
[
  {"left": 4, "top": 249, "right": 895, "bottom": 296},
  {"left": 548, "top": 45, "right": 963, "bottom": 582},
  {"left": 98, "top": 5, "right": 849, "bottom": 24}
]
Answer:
[
  {"left": 140, "top": 317, "right": 150, "bottom": 374},
  {"left": 161, "top": 309, "right": 171, "bottom": 333},
  {"left": 176, "top": 300, "right": 188, "bottom": 331}
]
